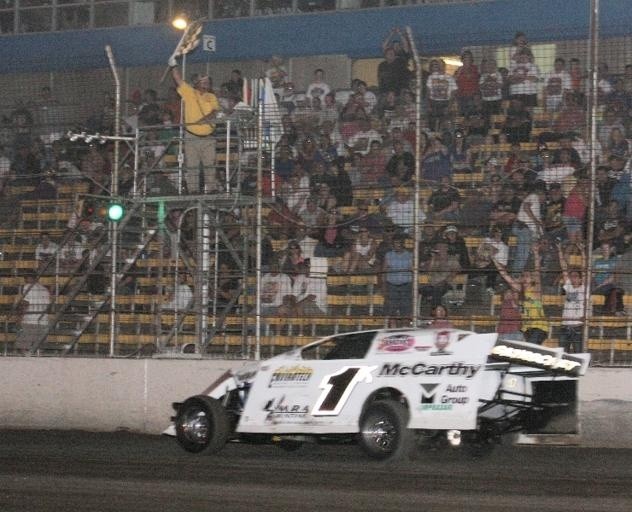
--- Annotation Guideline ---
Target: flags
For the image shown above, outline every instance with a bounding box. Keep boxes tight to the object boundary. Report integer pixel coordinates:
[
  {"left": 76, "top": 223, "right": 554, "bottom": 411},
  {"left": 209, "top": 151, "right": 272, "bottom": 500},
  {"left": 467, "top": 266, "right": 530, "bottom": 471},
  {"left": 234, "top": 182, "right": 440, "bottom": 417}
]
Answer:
[{"left": 174, "top": 23, "right": 203, "bottom": 56}]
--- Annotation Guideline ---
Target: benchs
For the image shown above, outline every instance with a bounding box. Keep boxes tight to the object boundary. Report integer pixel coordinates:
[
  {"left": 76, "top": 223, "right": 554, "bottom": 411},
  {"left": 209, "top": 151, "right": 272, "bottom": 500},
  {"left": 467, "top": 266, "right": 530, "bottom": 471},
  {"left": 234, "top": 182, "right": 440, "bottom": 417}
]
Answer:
[{"left": 0, "top": 75, "right": 632, "bottom": 356}]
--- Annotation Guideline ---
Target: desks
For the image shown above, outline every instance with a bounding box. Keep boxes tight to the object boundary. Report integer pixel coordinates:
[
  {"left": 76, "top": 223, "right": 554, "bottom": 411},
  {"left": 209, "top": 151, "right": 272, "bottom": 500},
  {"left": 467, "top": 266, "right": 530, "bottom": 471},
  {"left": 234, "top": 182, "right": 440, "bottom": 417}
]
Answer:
[
  {"left": 402, "top": 22, "right": 463, "bottom": 331},
  {"left": 168, "top": 15, "right": 195, "bottom": 196}
]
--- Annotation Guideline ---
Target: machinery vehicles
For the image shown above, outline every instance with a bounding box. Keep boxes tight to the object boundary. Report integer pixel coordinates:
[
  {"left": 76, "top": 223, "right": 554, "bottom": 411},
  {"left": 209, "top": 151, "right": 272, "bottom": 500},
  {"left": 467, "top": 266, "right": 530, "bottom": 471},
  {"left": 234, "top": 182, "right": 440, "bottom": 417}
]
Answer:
[{"left": 155, "top": 327, "right": 593, "bottom": 455}]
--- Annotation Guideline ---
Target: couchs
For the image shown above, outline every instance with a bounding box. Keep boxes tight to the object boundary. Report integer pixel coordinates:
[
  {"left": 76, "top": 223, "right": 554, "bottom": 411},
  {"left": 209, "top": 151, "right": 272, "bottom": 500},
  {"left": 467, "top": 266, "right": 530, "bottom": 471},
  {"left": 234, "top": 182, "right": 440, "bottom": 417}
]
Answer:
[{"left": 81, "top": 197, "right": 124, "bottom": 225}]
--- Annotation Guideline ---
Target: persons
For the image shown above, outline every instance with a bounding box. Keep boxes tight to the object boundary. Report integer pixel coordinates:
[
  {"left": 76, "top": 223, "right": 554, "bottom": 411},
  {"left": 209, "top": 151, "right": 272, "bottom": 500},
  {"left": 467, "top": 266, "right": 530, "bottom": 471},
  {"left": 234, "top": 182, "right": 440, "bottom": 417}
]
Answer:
[
  {"left": 167, "top": 56, "right": 220, "bottom": 193},
  {"left": 1, "top": 67, "right": 264, "bottom": 355},
  {"left": 258, "top": 32, "right": 630, "bottom": 365},
  {"left": 326, "top": 339, "right": 341, "bottom": 357}
]
